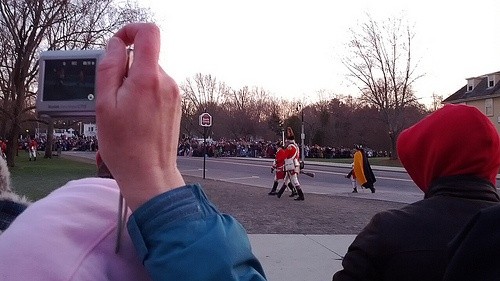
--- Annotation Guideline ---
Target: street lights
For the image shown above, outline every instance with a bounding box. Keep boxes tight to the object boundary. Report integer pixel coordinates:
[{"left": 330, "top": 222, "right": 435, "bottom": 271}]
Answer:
[{"left": 296, "top": 103, "right": 305, "bottom": 165}]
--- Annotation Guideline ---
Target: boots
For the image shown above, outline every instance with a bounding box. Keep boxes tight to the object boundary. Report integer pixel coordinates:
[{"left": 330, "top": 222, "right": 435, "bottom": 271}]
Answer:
[
  {"left": 294, "top": 189, "right": 304, "bottom": 200},
  {"left": 370, "top": 187, "right": 375, "bottom": 193},
  {"left": 352, "top": 187, "right": 358, "bottom": 193},
  {"left": 277, "top": 182, "right": 287, "bottom": 198},
  {"left": 269, "top": 182, "right": 278, "bottom": 195},
  {"left": 288, "top": 182, "right": 297, "bottom": 197}
]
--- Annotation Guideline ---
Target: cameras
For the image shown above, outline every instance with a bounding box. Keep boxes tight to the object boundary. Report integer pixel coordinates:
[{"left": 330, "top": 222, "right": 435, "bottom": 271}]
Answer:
[{"left": 36, "top": 47, "right": 133, "bottom": 122}]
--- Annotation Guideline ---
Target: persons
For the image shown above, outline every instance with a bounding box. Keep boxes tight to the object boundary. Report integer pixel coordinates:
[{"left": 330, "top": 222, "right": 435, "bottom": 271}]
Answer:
[
  {"left": 267, "top": 127, "right": 305, "bottom": 200},
  {"left": 94, "top": 22, "right": 268, "bottom": 281},
  {"left": 0, "top": 151, "right": 157, "bottom": 281},
  {"left": 345, "top": 144, "right": 377, "bottom": 194},
  {"left": 177, "top": 135, "right": 281, "bottom": 159},
  {"left": 332, "top": 103, "right": 500, "bottom": 281},
  {"left": 0, "top": 133, "right": 100, "bottom": 162},
  {"left": 297, "top": 143, "right": 391, "bottom": 159}
]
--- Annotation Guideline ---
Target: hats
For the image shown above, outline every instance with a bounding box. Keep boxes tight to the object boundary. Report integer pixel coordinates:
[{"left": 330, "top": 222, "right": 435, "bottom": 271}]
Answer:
[{"left": 285, "top": 127, "right": 295, "bottom": 143}]
